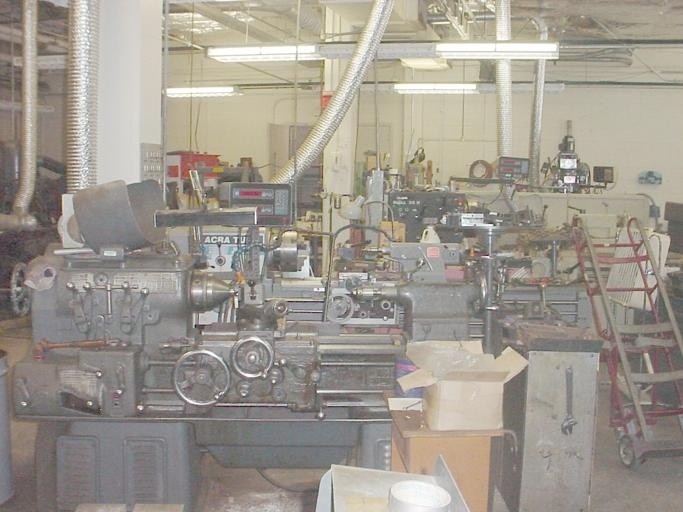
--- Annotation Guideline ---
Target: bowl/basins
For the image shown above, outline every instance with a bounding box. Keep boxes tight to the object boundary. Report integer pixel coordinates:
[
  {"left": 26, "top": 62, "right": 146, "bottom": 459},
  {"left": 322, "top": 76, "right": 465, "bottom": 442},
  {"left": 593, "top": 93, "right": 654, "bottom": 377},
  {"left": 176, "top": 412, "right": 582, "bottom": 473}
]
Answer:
[{"left": 387, "top": 480, "right": 453, "bottom": 512}]
